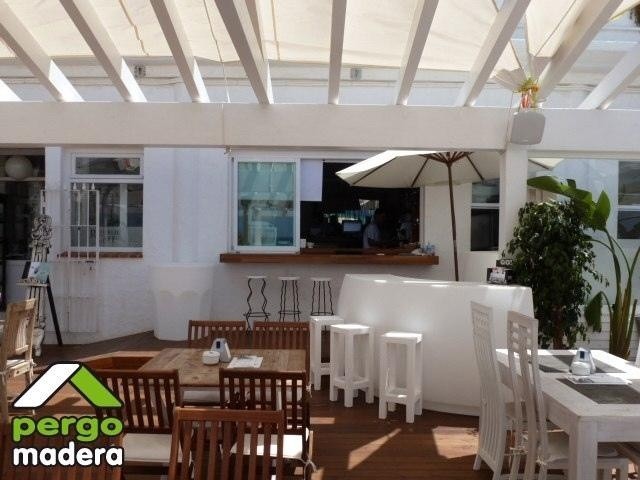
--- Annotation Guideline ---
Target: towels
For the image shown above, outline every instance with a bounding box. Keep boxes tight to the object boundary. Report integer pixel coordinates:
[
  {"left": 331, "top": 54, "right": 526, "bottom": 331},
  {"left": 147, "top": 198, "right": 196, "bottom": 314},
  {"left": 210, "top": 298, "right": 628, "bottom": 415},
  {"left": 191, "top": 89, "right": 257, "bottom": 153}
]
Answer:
[{"left": 228, "top": 353, "right": 264, "bottom": 370}]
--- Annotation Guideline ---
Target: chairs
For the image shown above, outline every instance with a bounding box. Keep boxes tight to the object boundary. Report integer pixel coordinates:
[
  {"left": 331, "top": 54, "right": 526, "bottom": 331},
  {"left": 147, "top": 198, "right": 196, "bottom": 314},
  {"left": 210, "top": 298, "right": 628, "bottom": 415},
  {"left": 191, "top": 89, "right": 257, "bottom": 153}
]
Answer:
[
  {"left": 187, "top": 319, "right": 247, "bottom": 348},
  {"left": 167, "top": 404, "right": 283, "bottom": 480},
  {"left": 501, "top": 308, "right": 630, "bottom": 480},
  {"left": 1, "top": 296, "right": 38, "bottom": 428},
  {"left": 250, "top": 322, "right": 307, "bottom": 349},
  {"left": 468, "top": 299, "right": 566, "bottom": 480},
  {"left": 89, "top": 368, "right": 190, "bottom": 480},
  {"left": 214, "top": 365, "right": 316, "bottom": 479},
  {"left": 7, "top": 415, "right": 124, "bottom": 476}
]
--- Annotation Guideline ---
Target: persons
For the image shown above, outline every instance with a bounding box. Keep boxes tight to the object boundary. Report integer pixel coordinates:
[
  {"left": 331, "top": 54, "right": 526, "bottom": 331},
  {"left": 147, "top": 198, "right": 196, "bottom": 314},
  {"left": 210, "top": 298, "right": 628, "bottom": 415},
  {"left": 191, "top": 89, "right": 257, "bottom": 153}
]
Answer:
[
  {"left": 363, "top": 208, "right": 389, "bottom": 250},
  {"left": 397, "top": 211, "right": 413, "bottom": 248}
]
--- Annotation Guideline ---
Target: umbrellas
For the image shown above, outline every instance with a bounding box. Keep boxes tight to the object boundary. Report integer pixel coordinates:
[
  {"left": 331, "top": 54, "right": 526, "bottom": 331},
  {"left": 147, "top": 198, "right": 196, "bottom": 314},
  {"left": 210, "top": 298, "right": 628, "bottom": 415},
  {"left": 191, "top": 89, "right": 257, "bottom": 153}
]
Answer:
[{"left": 337, "top": 147, "right": 565, "bottom": 285}]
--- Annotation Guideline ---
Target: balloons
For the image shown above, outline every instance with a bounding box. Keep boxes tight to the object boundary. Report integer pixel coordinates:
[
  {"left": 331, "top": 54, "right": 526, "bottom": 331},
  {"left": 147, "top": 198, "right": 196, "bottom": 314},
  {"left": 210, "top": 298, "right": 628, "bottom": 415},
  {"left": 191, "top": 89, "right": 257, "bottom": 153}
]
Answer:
[{"left": 5, "top": 156, "right": 32, "bottom": 179}]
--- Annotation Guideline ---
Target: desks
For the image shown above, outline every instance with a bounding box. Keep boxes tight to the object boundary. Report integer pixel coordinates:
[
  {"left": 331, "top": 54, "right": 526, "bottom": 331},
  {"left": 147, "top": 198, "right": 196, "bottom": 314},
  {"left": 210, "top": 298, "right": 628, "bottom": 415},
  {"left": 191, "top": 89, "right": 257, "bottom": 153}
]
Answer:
[
  {"left": 119, "top": 345, "right": 309, "bottom": 431},
  {"left": 10, "top": 429, "right": 316, "bottom": 480},
  {"left": 491, "top": 339, "right": 640, "bottom": 480},
  {"left": 336, "top": 274, "right": 537, "bottom": 418}
]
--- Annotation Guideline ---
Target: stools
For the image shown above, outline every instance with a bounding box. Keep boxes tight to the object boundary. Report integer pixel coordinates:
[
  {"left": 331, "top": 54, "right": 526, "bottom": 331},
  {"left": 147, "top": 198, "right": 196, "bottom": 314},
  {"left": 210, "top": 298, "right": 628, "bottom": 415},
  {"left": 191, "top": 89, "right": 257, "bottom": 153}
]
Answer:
[
  {"left": 244, "top": 274, "right": 271, "bottom": 335},
  {"left": 377, "top": 331, "right": 427, "bottom": 425},
  {"left": 277, "top": 276, "right": 303, "bottom": 334},
  {"left": 310, "top": 276, "right": 335, "bottom": 320},
  {"left": 308, "top": 314, "right": 342, "bottom": 391},
  {"left": 329, "top": 322, "right": 376, "bottom": 407}
]
